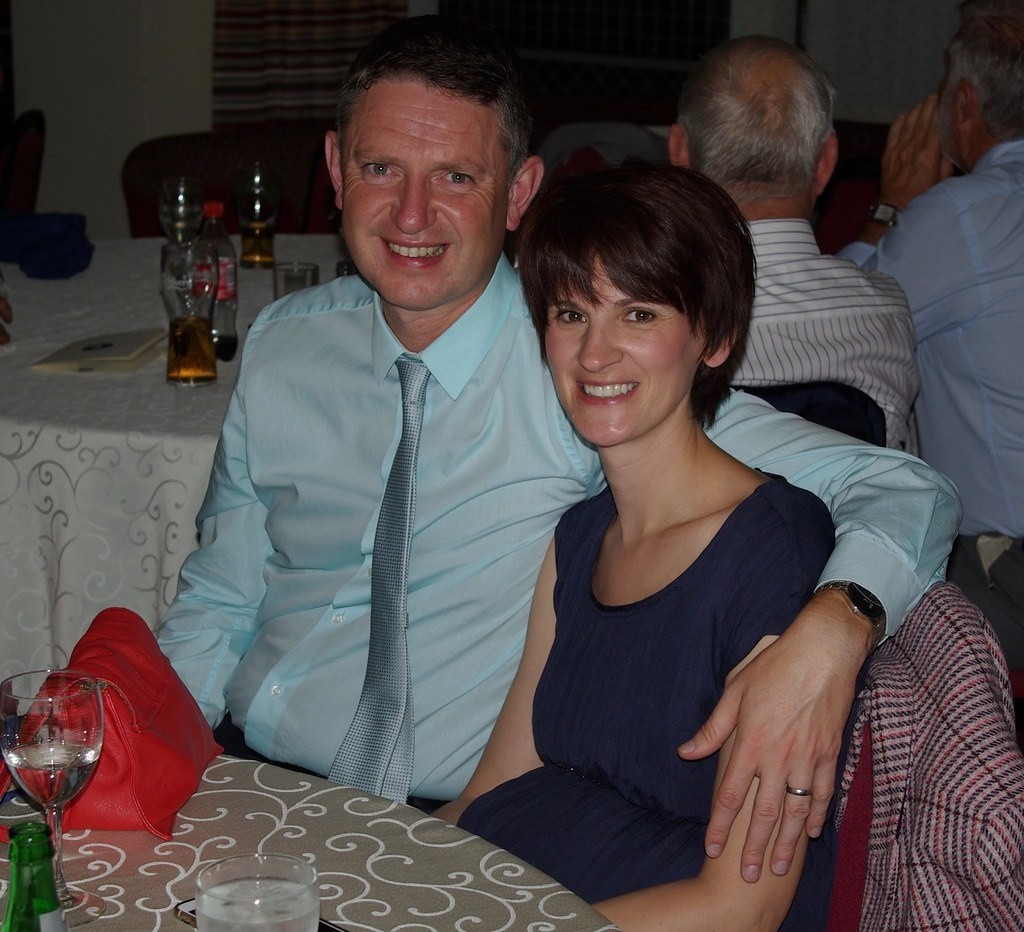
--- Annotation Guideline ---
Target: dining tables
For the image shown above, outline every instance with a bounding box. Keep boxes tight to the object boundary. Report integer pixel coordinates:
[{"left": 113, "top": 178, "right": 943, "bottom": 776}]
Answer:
[
  {"left": 1, "top": 232, "right": 341, "bottom": 720},
  {"left": 0, "top": 754, "right": 625, "bottom": 932}
]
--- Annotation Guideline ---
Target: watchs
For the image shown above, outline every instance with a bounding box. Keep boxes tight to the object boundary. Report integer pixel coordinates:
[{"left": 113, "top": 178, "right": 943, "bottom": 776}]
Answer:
[
  {"left": 869, "top": 198, "right": 902, "bottom": 228},
  {"left": 816, "top": 580, "right": 886, "bottom": 655}
]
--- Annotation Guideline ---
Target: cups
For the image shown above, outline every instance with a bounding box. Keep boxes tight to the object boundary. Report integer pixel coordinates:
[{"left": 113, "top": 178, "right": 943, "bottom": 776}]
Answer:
[
  {"left": 273, "top": 262, "right": 318, "bottom": 304},
  {"left": 231, "top": 158, "right": 280, "bottom": 270},
  {"left": 196, "top": 852, "right": 320, "bottom": 931},
  {"left": 159, "top": 242, "right": 218, "bottom": 387}
]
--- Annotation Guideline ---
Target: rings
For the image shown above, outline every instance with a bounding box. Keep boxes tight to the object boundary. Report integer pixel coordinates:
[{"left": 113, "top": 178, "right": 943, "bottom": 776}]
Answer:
[{"left": 786, "top": 785, "right": 813, "bottom": 797}]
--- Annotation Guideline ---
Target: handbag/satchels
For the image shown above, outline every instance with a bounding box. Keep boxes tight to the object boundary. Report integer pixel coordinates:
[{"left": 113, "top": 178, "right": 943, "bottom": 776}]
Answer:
[{"left": 0, "top": 607, "right": 223, "bottom": 842}]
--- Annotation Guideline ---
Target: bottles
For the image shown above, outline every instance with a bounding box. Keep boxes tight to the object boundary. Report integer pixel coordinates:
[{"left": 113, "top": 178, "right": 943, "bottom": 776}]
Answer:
[
  {"left": 1, "top": 819, "right": 71, "bottom": 932},
  {"left": 186, "top": 201, "right": 240, "bottom": 362},
  {"left": 336, "top": 261, "right": 356, "bottom": 278}
]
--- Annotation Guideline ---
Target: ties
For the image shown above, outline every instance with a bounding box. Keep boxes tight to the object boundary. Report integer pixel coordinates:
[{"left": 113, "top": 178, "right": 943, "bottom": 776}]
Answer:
[{"left": 328, "top": 362, "right": 433, "bottom": 802}]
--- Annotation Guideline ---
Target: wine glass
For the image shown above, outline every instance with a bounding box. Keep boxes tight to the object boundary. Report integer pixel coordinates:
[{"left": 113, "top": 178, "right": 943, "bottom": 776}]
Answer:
[
  {"left": 158, "top": 161, "right": 206, "bottom": 297},
  {"left": 0, "top": 670, "right": 108, "bottom": 929}
]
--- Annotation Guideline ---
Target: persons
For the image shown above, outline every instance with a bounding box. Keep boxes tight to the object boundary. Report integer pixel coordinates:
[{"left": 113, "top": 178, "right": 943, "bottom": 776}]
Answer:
[
  {"left": 824, "top": 18, "right": 1022, "bottom": 723},
  {"left": 419, "top": 155, "right": 872, "bottom": 932},
  {"left": 667, "top": 33, "right": 923, "bottom": 452},
  {"left": 149, "top": 14, "right": 965, "bottom": 882}
]
